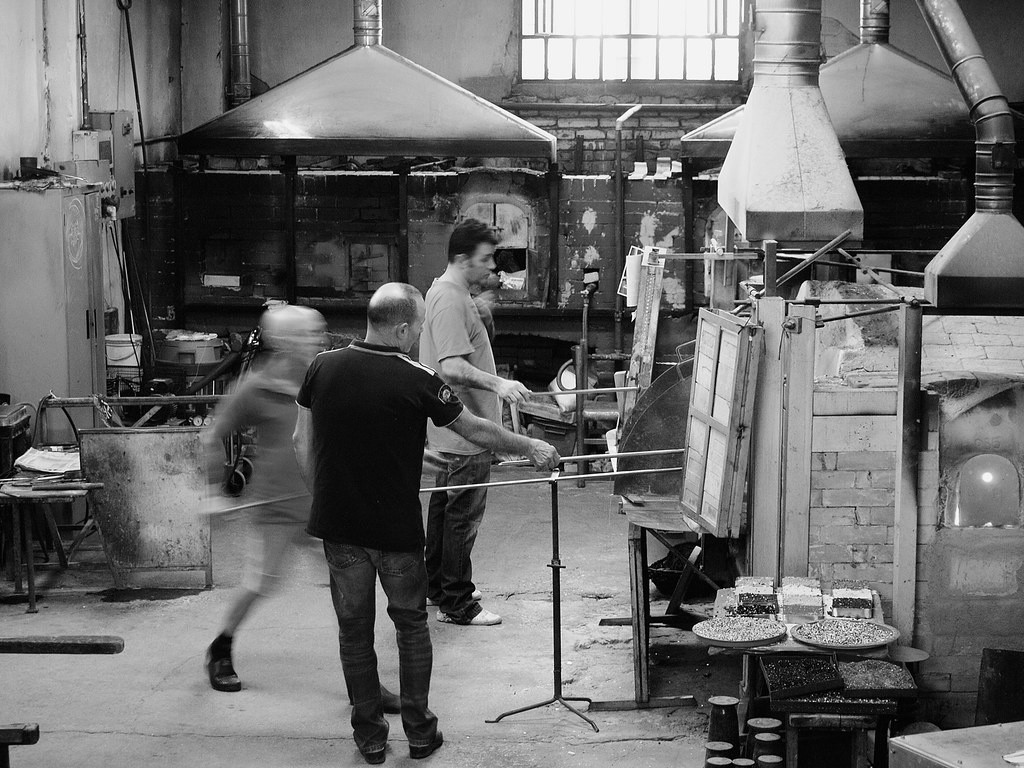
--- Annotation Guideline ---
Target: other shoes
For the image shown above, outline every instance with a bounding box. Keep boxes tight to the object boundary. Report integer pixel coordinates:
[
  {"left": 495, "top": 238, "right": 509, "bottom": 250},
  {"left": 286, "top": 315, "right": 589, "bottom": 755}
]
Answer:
[
  {"left": 410, "top": 732, "right": 443, "bottom": 758},
  {"left": 426, "top": 588, "right": 482, "bottom": 606},
  {"left": 437, "top": 610, "right": 502, "bottom": 625},
  {"left": 350, "top": 681, "right": 402, "bottom": 714},
  {"left": 207, "top": 635, "right": 241, "bottom": 691},
  {"left": 359, "top": 743, "right": 387, "bottom": 764}
]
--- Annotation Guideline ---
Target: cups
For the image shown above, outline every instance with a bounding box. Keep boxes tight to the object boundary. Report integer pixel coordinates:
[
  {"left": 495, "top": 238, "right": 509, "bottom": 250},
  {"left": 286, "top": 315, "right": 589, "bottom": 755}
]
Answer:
[{"left": 20, "top": 157, "right": 37, "bottom": 177}]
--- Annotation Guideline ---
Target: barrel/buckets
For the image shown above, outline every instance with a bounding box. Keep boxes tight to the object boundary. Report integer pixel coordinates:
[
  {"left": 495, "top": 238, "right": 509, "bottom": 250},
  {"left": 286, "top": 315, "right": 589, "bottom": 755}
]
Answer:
[{"left": 105, "top": 334, "right": 142, "bottom": 366}]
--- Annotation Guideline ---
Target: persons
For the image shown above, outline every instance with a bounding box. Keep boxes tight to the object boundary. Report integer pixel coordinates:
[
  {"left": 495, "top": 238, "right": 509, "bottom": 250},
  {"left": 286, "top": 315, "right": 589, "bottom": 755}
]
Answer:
[
  {"left": 418, "top": 218, "right": 532, "bottom": 626},
  {"left": 198, "top": 306, "right": 401, "bottom": 713},
  {"left": 292, "top": 282, "right": 561, "bottom": 764}
]
